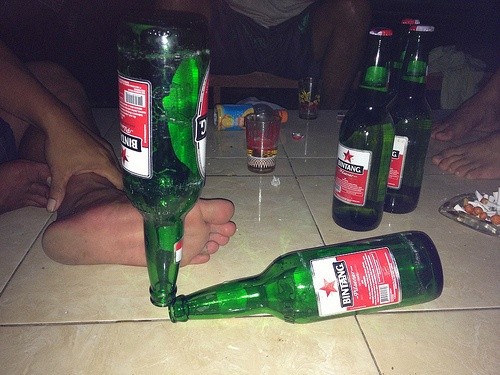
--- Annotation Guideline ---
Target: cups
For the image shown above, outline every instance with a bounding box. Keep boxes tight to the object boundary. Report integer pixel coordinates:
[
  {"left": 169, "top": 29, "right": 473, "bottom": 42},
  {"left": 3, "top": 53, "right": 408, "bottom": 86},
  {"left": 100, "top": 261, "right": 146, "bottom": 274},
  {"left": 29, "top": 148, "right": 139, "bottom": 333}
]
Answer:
[
  {"left": 246, "top": 112, "right": 281, "bottom": 173},
  {"left": 298, "top": 78, "right": 322, "bottom": 119}
]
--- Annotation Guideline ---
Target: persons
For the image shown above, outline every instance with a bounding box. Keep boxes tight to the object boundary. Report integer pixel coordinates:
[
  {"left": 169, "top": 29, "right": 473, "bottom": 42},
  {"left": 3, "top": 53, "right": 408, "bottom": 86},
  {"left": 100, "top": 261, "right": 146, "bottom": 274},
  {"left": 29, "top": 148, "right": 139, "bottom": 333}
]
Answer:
[
  {"left": 0, "top": 40, "right": 236, "bottom": 267},
  {"left": 429, "top": 67, "right": 500, "bottom": 180},
  {"left": 153, "top": 0, "right": 373, "bottom": 125}
]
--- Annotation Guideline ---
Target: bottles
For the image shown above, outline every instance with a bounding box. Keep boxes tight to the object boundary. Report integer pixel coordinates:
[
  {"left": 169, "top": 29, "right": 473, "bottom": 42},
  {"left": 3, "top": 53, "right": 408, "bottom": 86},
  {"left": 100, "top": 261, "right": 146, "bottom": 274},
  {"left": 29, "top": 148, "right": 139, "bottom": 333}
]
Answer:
[
  {"left": 169, "top": 230, "right": 443, "bottom": 322},
  {"left": 332, "top": 29, "right": 395, "bottom": 231},
  {"left": 387, "top": 17, "right": 420, "bottom": 99},
  {"left": 117, "top": 10, "right": 211, "bottom": 306},
  {"left": 383, "top": 25, "right": 433, "bottom": 213}
]
